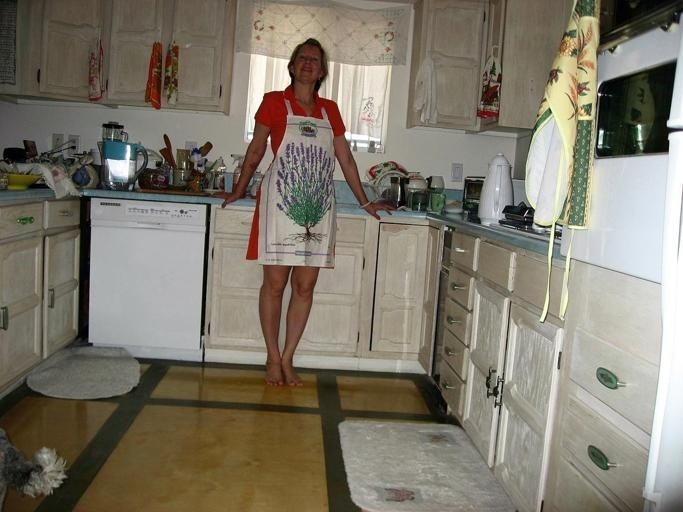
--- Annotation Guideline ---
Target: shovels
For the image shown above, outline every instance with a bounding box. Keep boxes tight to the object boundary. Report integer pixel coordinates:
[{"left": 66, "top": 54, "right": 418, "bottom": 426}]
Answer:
[
  {"left": 159, "top": 147, "right": 177, "bottom": 169},
  {"left": 188, "top": 141, "right": 213, "bottom": 170}
]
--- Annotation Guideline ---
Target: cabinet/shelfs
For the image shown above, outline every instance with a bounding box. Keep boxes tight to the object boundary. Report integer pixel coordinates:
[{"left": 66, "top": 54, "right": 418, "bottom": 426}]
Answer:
[
  {"left": 460, "top": 240, "right": 569, "bottom": 511},
  {"left": 0, "top": 0, "right": 237, "bottom": 117},
  {"left": 548, "top": 328, "right": 658, "bottom": 511},
  {"left": 369, "top": 221, "right": 480, "bottom": 421},
  {"left": 210, "top": 207, "right": 366, "bottom": 354},
  {"left": 405, "top": 0, "right": 577, "bottom": 140},
  {"left": 0, "top": 199, "right": 81, "bottom": 388}
]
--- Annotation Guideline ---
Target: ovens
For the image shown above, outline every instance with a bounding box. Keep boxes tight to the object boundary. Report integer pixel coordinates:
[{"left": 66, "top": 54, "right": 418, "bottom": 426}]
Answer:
[{"left": 558, "top": 11, "right": 683, "bottom": 285}]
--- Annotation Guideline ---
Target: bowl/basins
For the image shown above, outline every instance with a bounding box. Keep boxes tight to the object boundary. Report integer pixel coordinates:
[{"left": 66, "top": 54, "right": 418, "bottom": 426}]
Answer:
[{"left": 5, "top": 173, "right": 42, "bottom": 191}]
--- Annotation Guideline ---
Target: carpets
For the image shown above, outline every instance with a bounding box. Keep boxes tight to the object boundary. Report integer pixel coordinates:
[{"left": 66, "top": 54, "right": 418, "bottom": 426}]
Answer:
[
  {"left": 25, "top": 346, "right": 142, "bottom": 401},
  {"left": 338, "top": 418, "right": 519, "bottom": 512}
]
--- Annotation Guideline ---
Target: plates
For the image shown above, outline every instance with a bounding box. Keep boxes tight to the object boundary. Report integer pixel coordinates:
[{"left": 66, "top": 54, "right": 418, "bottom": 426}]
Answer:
[{"left": 444, "top": 208, "right": 464, "bottom": 214}]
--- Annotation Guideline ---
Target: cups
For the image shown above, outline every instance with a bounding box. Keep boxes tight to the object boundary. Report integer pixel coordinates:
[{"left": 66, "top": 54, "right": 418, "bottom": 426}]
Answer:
[
  {"left": 390, "top": 176, "right": 405, "bottom": 209},
  {"left": 431, "top": 193, "right": 446, "bottom": 212},
  {"left": 411, "top": 192, "right": 427, "bottom": 212}
]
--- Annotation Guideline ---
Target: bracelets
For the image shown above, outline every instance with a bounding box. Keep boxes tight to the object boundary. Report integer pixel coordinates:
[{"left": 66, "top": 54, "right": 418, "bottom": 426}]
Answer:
[{"left": 358, "top": 201, "right": 371, "bottom": 209}]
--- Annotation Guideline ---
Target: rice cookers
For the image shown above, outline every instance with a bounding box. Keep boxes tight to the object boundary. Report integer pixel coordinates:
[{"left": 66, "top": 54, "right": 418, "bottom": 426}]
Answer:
[{"left": 134, "top": 148, "right": 162, "bottom": 187}]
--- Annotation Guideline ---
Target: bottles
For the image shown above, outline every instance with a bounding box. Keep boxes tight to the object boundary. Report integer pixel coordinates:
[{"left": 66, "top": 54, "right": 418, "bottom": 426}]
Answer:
[{"left": 253, "top": 169, "right": 264, "bottom": 197}]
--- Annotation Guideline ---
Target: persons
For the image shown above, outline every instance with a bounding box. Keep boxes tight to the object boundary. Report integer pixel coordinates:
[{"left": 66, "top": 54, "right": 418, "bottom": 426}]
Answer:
[{"left": 211, "top": 37, "right": 401, "bottom": 387}]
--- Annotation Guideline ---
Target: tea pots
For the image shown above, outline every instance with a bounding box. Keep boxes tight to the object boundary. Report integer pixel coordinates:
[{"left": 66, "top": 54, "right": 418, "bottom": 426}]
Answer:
[
  {"left": 96, "top": 139, "right": 148, "bottom": 191},
  {"left": 99, "top": 121, "right": 129, "bottom": 144}
]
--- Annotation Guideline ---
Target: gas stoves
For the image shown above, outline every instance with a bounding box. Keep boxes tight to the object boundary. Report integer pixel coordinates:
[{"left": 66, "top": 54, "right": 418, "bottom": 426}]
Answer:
[{"left": 498, "top": 200, "right": 535, "bottom": 231}]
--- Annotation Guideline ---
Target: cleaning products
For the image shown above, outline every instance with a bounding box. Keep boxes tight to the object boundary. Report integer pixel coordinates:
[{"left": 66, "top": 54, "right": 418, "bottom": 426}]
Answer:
[{"left": 231, "top": 153, "right": 247, "bottom": 199}]
